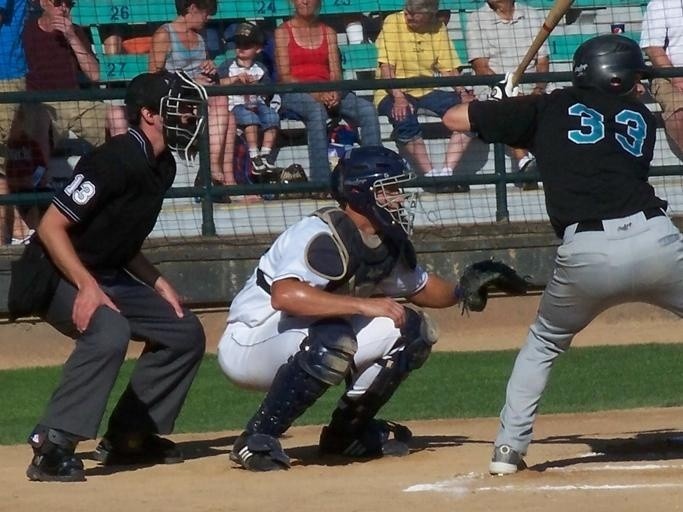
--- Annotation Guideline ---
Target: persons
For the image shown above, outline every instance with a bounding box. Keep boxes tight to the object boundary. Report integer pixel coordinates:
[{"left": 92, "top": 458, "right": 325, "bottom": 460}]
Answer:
[
  {"left": 442, "top": 35, "right": 683, "bottom": 476},
  {"left": 217, "top": 146, "right": 528, "bottom": 473},
  {"left": 639, "top": 0, "right": 683, "bottom": 162},
  {"left": 149, "top": 1, "right": 551, "bottom": 202},
  {"left": 1, "top": 1, "right": 128, "bottom": 246},
  {"left": 8, "top": 72, "right": 207, "bottom": 482}
]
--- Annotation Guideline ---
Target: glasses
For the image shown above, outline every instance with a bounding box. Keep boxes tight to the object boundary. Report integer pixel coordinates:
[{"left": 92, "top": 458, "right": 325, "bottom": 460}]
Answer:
[{"left": 51, "top": 1, "right": 76, "bottom": 9}]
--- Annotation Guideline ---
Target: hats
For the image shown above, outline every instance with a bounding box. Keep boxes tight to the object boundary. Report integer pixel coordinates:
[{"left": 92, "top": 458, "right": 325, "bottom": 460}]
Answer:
[{"left": 224, "top": 21, "right": 265, "bottom": 48}]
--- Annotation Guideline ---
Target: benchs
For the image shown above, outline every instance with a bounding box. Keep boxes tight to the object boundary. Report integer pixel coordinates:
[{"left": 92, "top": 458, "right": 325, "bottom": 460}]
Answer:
[{"left": 67, "top": 0, "right": 683, "bottom": 193}]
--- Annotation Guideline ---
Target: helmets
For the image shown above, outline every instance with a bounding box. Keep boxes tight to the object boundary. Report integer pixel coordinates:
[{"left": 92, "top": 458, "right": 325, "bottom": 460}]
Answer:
[
  {"left": 329, "top": 145, "right": 424, "bottom": 236},
  {"left": 124, "top": 66, "right": 209, "bottom": 168},
  {"left": 572, "top": 34, "right": 643, "bottom": 98}
]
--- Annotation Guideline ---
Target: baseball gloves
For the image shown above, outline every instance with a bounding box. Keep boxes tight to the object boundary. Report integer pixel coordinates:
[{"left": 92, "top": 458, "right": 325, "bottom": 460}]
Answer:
[{"left": 455, "top": 261, "right": 534, "bottom": 319}]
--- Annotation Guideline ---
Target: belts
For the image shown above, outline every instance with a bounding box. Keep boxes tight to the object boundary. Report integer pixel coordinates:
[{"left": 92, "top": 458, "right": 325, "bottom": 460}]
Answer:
[{"left": 575, "top": 208, "right": 666, "bottom": 233}]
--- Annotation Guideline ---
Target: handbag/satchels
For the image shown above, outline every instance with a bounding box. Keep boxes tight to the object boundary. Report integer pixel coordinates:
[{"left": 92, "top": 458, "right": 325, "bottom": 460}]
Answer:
[{"left": 261, "top": 163, "right": 311, "bottom": 200}]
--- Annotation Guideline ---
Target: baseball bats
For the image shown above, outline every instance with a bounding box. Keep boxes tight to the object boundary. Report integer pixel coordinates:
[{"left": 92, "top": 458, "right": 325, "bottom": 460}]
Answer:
[{"left": 513, "top": 0, "right": 575, "bottom": 89}]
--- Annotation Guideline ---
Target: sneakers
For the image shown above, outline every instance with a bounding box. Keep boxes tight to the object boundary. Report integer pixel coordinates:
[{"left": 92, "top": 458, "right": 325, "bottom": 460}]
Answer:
[
  {"left": 26, "top": 447, "right": 87, "bottom": 482},
  {"left": 249, "top": 154, "right": 277, "bottom": 177},
  {"left": 520, "top": 158, "right": 540, "bottom": 190},
  {"left": 229, "top": 431, "right": 277, "bottom": 471},
  {"left": 425, "top": 183, "right": 470, "bottom": 193},
  {"left": 316, "top": 420, "right": 412, "bottom": 464},
  {"left": 96, "top": 436, "right": 186, "bottom": 466},
  {"left": 490, "top": 444, "right": 527, "bottom": 474}
]
